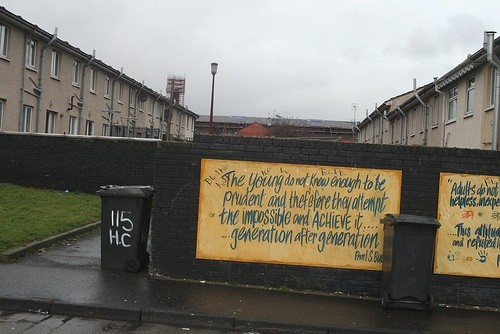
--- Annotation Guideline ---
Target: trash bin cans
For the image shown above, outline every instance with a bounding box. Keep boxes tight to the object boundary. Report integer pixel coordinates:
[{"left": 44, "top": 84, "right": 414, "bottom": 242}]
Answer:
[
  {"left": 380, "top": 213, "right": 442, "bottom": 308},
  {"left": 96, "top": 184, "right": 151, "bottom": 272}
]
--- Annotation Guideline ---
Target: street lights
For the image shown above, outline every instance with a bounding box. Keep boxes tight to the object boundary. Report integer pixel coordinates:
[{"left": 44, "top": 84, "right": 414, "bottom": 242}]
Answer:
[{"left": 208, "top": 62, "right": 217, "bottom": 136}]
